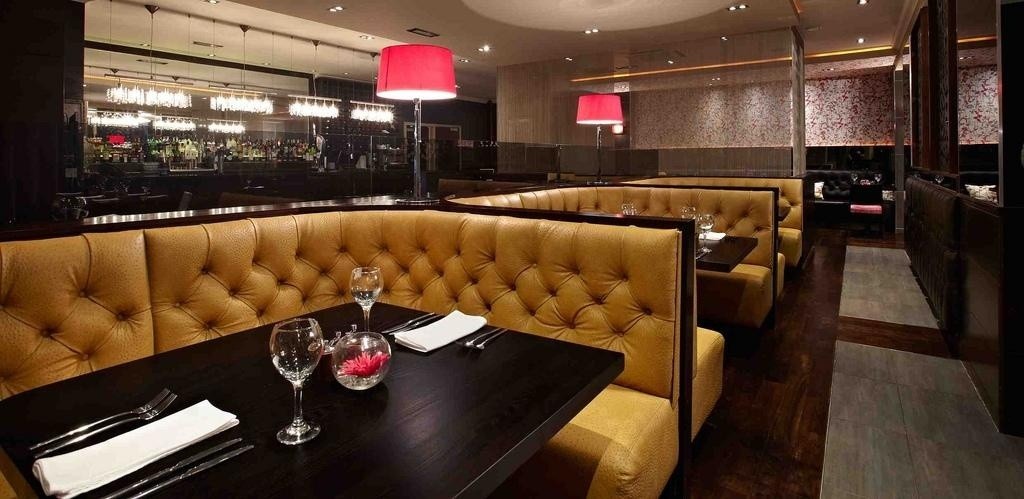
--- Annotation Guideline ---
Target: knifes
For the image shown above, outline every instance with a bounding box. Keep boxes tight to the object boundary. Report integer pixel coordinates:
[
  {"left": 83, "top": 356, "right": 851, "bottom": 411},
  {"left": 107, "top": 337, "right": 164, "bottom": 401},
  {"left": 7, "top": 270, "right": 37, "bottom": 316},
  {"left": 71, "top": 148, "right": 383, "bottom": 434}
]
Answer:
[
  {"left": 103, "top": 438, "right": 255, "bottom": 499},
  {"left": 381, "top": 312, "right": 436, "bottom": 334},
  {"left": 388, "top": 314, "right": 444, "bottom": 335},
  {"left": 696, "top": 251, "right": 707, "bottom": 260}
]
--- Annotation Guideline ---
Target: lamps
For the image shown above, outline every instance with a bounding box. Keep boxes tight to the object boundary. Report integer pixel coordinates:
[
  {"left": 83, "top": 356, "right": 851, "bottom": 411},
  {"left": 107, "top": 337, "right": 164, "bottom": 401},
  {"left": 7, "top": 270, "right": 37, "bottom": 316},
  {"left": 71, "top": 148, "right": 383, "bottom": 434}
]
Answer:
[
  {"left": 575, "top": 93, "right": 624, "bottom": 185},
  {"left": 377, "top": 44, "right": 457, "bottom": 203},
  {"left": 85, "top": 69, "right": 247, "bottom": 135},
  {"left": 105, "top": 4, "right": 394, "bottom": 125}
]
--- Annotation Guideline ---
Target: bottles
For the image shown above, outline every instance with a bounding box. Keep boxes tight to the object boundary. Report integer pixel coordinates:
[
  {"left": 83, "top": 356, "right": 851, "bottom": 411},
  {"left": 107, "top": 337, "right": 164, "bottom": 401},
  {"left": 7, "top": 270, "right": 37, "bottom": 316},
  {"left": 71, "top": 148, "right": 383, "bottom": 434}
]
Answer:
[
  {"left": 102, "top": 138, "right": 142, "bottom": 163},
  {"left": 225, "top": 135, "right": 316, "bottom": 162},
  {"left": 146, "top": 134, "right": 224, "bottom": 169}
]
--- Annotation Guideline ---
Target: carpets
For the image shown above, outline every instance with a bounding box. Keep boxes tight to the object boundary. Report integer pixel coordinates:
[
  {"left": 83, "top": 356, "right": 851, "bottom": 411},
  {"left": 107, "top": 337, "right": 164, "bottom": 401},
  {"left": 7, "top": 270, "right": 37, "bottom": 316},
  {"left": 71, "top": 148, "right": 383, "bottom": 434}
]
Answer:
[
  {"left": 838, "top": 244, "right": 942, "bottom": 329},
  {"left": 819, "top": 337, "right": 1023, "bottom": 499}
]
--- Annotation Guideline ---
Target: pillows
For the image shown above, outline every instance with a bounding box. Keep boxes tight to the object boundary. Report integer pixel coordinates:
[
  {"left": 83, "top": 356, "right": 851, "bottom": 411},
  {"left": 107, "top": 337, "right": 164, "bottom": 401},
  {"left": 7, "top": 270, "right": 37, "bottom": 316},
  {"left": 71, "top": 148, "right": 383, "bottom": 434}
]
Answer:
[
  {"left": 813, "top": 181, "right": 826, "bottom": 201},
  {"left": 962, "top": 182, "right": 1001, "bottom": 205}
]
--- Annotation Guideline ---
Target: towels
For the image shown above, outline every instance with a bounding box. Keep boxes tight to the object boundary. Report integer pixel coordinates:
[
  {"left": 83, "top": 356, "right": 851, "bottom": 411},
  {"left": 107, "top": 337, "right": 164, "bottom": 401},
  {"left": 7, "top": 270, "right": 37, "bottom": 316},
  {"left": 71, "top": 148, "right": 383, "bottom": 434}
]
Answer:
[
  {"left": 702, "top": 232, "right": 728, "bottom": 241},
  {"left": 33, "top": 398, "right": 242, "bottom": 499},
  {"left": 392, "top": 310, "right": 487, "bottom": 354}
]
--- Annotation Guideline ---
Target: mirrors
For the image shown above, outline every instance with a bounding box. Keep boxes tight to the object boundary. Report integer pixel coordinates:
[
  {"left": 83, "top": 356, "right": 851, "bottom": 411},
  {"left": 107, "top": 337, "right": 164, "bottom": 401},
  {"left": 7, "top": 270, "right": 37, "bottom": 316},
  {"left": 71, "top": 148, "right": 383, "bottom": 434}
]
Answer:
[
  {"left": 950, "top": 1, "right": 1006, "bottom": 205},
  {"left": 84, "top": 39, "right": 314, "bottom": 163}
]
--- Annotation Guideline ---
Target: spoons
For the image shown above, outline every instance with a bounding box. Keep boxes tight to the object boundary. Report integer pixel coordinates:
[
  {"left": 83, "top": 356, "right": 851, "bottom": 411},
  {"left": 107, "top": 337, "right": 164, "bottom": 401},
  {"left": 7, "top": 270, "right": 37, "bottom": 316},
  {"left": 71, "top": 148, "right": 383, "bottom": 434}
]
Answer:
[{"left": 454, "top": 327, "right": 502, "bottom": 349}]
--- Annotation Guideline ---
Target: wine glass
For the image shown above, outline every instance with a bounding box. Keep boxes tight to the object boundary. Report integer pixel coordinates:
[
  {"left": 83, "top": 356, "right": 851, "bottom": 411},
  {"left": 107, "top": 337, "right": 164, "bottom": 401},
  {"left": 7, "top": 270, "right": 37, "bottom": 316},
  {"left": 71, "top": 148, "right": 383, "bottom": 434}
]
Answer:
[
  {"left": 679, "top": 205, "right": 714, "bottom": 254},
  {"left": 349, "top": 267, "right": 384, "bottom": 333},
  {"left": 914, "top": 174, "right": 920, "bottom": 178},
  {"left": 270, "top": 318, "right": 324, "bottom": 447},
  {"left": 622, "top": 204, "right": 635, "bottom": 215},
  {"left": 851, "top": 174, "right": 858, "bottom": 184},
  {"left": 935, "top": 175, "right": 944, "bottom": 185},
  {"left": 874, "top": 174, "right": 882, "bottom": 183}
]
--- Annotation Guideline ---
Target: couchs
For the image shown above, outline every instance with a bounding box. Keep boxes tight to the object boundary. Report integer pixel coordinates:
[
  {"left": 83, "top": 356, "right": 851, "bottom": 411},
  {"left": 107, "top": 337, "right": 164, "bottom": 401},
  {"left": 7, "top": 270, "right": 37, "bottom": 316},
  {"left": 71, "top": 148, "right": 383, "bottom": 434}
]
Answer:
[
  {"left": 803, "top": 169, "right": 884, "bottom": 228},
  {"left": 1, "top": 202, "right": 689, "bottom": 499},
  {"left": 630, "top": 173, "right": 817, "bottom": 297},
  {"left": 437, "top": 181, "right": 781, "bottom": 453}
]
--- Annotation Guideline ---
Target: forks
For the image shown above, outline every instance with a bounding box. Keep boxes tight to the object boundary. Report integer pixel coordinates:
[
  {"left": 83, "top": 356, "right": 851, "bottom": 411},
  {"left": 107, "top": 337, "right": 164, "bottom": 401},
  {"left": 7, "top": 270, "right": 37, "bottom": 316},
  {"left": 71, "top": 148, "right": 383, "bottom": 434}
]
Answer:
[
  {"left": 28, "top": 388, "right": 177, "bottom": 459},
  {"left": 468, "top": 328, "right": 510, "bottom": 350}
]
